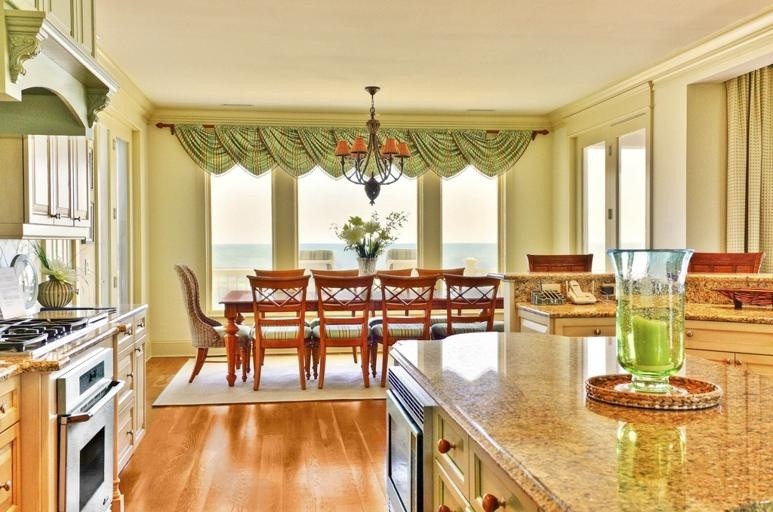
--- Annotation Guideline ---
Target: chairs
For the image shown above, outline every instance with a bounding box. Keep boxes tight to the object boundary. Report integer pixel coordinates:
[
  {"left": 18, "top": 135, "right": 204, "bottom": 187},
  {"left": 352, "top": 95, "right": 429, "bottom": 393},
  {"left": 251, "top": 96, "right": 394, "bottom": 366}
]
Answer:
[
  {"left": 173, "top": 262, "right": 253, "bottom": 382},
  {"left": 687, "top": 251, "right": 766, "bottom": 274},
  {"left": 523, "top": 251, "right": 594, "bottom": 273},
  {"left": 219, "top": 261, "right": 500, "bottom": 392}
]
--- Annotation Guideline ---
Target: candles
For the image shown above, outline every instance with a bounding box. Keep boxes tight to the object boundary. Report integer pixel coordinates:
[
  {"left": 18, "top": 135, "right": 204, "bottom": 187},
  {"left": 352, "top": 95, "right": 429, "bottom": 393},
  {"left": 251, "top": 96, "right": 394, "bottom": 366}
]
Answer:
[{"left": 631, "top": 313, "right": 669, "bottom": 368}]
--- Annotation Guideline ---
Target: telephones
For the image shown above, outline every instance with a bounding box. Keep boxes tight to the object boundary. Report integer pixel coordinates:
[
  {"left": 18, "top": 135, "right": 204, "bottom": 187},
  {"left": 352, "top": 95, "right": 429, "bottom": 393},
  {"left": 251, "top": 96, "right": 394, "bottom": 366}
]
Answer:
[{"left": 568, "top": 280, "right": 596, "bottom": 304}]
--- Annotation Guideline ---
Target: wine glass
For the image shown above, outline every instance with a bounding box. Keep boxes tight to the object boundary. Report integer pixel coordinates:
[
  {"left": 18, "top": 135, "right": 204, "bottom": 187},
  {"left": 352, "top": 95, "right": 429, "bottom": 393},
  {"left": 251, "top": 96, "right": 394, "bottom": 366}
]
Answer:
[{"left": 611, "top": 245, "right": 695, "bottom": 396}]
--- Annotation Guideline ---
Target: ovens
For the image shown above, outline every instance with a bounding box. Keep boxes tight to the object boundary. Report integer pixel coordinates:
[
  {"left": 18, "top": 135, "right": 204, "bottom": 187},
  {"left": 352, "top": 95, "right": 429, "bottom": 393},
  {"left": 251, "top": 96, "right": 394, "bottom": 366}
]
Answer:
[{"left": 58, "top": 346, "right": 126, "bottom": 512}]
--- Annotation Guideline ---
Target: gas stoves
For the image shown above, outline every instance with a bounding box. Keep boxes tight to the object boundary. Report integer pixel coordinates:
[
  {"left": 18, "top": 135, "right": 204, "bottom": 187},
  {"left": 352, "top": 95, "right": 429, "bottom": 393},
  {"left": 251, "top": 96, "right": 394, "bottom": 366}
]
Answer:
[{"left": 0, "top": 309, "right": 120, "bottom": 370}]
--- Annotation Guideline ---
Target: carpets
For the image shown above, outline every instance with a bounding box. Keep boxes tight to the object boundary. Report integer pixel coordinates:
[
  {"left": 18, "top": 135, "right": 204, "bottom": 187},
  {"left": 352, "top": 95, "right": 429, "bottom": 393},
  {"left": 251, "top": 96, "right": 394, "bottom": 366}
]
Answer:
[{"left": 150, "top": 352, "right": 394, "bottom": 409}]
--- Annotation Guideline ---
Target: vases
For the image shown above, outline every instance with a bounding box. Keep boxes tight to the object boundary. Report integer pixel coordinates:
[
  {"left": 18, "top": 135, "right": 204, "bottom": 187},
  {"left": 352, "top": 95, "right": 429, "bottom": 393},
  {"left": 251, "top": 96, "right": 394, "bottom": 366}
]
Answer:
[
  {"left": 34, "top": 273, "right": 74, "bottom": 308},
  {"left": 355, "top": 256, "right": 379, "bottom": 277}
]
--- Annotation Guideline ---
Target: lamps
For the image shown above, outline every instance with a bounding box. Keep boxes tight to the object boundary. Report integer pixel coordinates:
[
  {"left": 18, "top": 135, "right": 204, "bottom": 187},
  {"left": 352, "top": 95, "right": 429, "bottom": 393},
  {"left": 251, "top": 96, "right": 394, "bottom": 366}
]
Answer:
[{"left": 333, "top": 85, "right": 412, "bottom": 207}]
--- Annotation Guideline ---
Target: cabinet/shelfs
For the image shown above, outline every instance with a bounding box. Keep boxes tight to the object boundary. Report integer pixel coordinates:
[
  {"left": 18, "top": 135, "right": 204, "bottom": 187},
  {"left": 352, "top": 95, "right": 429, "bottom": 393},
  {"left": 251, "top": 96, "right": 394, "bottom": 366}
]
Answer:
[
  {"left": 0, "top": 0, "right": 122, "bottom": 245},
  {"left": 516, "top": 310, "right": 616, "bottom": 336},
  {"left": 134, "top": 308, "right": 147, "bottom": 454},
  {"left": 111, "top": 315, "right": 135, "bottom": 512},
  {"left": 0, "top": 373, "right": 22, "bottom": 512},
  {"left": 681, "top": 319, "right": 772, "bottom": 373}
]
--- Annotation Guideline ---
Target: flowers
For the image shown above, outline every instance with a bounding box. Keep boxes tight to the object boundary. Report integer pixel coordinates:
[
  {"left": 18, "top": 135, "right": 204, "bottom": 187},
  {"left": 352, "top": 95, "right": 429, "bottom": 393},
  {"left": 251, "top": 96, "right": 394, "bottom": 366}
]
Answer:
[
  {"left": 333, "top": 209, "right": 408, "bottom": 273},
  {"left": 25, "top": 238, "right": 92, "bottom": 287}
]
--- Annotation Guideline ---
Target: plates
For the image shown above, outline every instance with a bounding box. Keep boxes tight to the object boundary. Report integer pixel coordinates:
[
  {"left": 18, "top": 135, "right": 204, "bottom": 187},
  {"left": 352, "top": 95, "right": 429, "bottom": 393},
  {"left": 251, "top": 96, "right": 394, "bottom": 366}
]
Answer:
[{"left": 12, "top": 255, "right": 38, "bottom": 307}]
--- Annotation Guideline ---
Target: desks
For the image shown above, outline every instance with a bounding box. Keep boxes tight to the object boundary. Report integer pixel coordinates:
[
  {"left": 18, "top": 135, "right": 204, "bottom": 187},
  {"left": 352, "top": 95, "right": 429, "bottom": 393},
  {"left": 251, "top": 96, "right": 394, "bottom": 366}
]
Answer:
[{"left": 383, "top": 330, "right": 772, "bottom": 512}]
